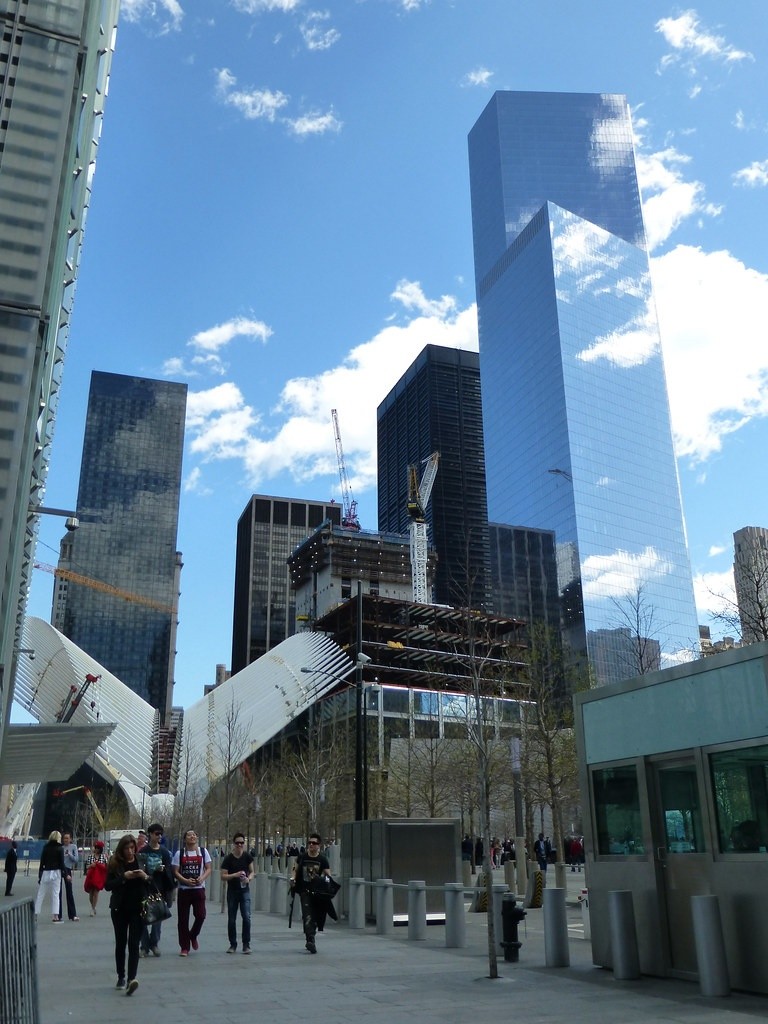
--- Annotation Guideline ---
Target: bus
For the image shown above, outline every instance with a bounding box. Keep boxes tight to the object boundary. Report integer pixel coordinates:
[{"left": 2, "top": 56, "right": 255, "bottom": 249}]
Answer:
[{"left": 98, "top": 830, "right": 145, "bottom": 859}]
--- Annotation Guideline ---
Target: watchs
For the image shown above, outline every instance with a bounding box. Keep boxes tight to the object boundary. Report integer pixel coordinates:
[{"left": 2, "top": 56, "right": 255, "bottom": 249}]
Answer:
[{"left": 144, "top": 876, "right": 149, "bottom": 881}]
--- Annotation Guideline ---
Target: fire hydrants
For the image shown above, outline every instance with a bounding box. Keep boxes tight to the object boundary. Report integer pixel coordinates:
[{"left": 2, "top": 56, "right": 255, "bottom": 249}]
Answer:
[{"left": 500, "top": 889, "right": 527, "bottom": 961}]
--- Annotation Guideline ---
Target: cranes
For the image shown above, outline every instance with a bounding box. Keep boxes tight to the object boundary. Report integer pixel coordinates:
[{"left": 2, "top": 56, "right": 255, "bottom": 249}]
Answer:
[
  {"left": 407, "top": 451, "right": 439, "bottom": 603},
  {"left": 331, "top": 409, "right": 360, "bottom": 528}
]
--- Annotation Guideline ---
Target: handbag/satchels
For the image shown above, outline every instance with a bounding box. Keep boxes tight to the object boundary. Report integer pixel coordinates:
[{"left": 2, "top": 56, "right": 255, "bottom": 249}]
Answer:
[{"left": 139, "top": 880, "right": 172, "bottom": 925}]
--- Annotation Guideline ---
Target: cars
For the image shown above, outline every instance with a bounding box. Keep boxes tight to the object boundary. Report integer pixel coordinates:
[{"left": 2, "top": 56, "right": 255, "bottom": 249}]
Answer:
[
  {"left": 610, "top": 844, "right": 627, "bottom": 854},
  {"left": 78, "top": 847, "right": 91, "bottom": 861},
  {"left": 669, "top": 842, "right": 694, "bottom": 853}
]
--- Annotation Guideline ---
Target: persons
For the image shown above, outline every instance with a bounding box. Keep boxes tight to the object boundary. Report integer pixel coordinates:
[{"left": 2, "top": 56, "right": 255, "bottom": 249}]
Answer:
[
  {"left": 250, "top": 847, "right": 256, "bottom": 857},
  {"left": 623, "top": 825, "right": 634, "bottom": 849},
  {"left": 135, "top": 824, "right": 176, "bottom": 958},
  {"left": 265, "top": 845, "right": 274, "bottom": 866},
  {"left": 214, "top": 847, "right": 225, "bottom": 862},
  {"left": 274, "top": 840, "right": 305, "bottom": 869},
  {"left": 534, "top": 833, "right": 551, "bottom": 874},
  {"left": 59, "top": 831, "right": 80, "bottom": 924},
  {"left": 563, "top": 832, "right": 586, "bottom": 872},
  {"left": 33, "top": 831, "right": 66, "bottom": 923},
  {"left": 729, "top": 818, "right": 761, "bottom": 853},
  {"left": 324, "top": 837, "right": 336, "bottom": 857},
  {"left": 4, "top": 841, "right": 19, "bottom": 896},
  {"left": 84, "top": 842, "right": 109, "bottom": 917},
  {"left": 220, "top": 832, "right": 255, "bottom": 954},
  {"left": 490, "top": 836, "right": 516, "bottom": 870},
  {"left": 103, "top": 834, "right": 159, "bottom": 996},
  {"left": 172, "top": 829, "right": 212, "bottom": 957},
  {"left": 290, "top": 834, "right": 332, "bottom": 953},
  {"left": 461, "top": 834, "right": 484, "bottom": 866}
]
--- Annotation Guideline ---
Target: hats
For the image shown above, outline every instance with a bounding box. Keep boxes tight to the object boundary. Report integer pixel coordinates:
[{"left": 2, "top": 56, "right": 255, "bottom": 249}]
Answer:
[{"left": 96, "top": 841, "right": 104, "bottom": 846}]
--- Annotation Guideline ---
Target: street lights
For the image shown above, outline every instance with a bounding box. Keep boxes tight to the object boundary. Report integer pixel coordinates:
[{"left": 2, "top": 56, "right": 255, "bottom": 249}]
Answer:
[
  {"left": 510, "top": 738, "right": 527, "bottom": 895},
  {"left": 300, "top": 579, "right": 362, "bottom": 821},
  {"left": 116, "top": 780, "right": 145, "bottom": 829}
]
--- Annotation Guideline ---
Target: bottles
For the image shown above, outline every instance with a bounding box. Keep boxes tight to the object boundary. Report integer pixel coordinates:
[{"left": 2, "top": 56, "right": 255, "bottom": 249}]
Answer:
[{"left": 240, "top": 871, "right": 247, "bottom": 888}]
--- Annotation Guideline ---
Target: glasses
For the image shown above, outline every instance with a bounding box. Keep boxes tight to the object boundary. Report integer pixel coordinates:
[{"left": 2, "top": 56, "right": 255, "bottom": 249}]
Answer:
[
  {"left": 154, "top": 832, "right": 163, "bottom": 836},
  {"left": 234, "top": 841, "right": 244, "bottom": 845},
  {"left": 308, "top": 841, "right": 319, "bottom": 845}
]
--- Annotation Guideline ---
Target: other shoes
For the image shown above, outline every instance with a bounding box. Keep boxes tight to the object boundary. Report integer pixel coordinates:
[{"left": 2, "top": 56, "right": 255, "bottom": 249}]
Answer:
[
  {"left": 126, "top": 979, "right": 138, "bottom": 995},
  {"left": 227, "top": 946, "right": 236, "bottom": 953},
  {"left": 5, "top": 893, "right": 13, "bottom": 896},
  {"left": 148, "top": 943, "right": 159, "bottom": 956},
  {"left": 116, "top": 979, "right": 125, "bottom": 989},
  {"left": 192, "top": 939, "right": 198, "bottom": 950},
  {"left": 69, "top": 916, "right": 79, "bottom": 921},
  {"left": 243, "top": 945, "right": 252, "bottom": 954},
  {"left": 306, "top": 941, "right": 317, "bottom": 953},
  {"left": 139, "top": 949, "right": 148, "bottom": 957},
  {"left": 180, "top": 948, "right": 188, "bottom": 956},
  {"left": 90, "top": 910, "right": 94, "bottom": 917},
  {"left": 53, "top": 918, "right": 64, "bottom": 923}
]
